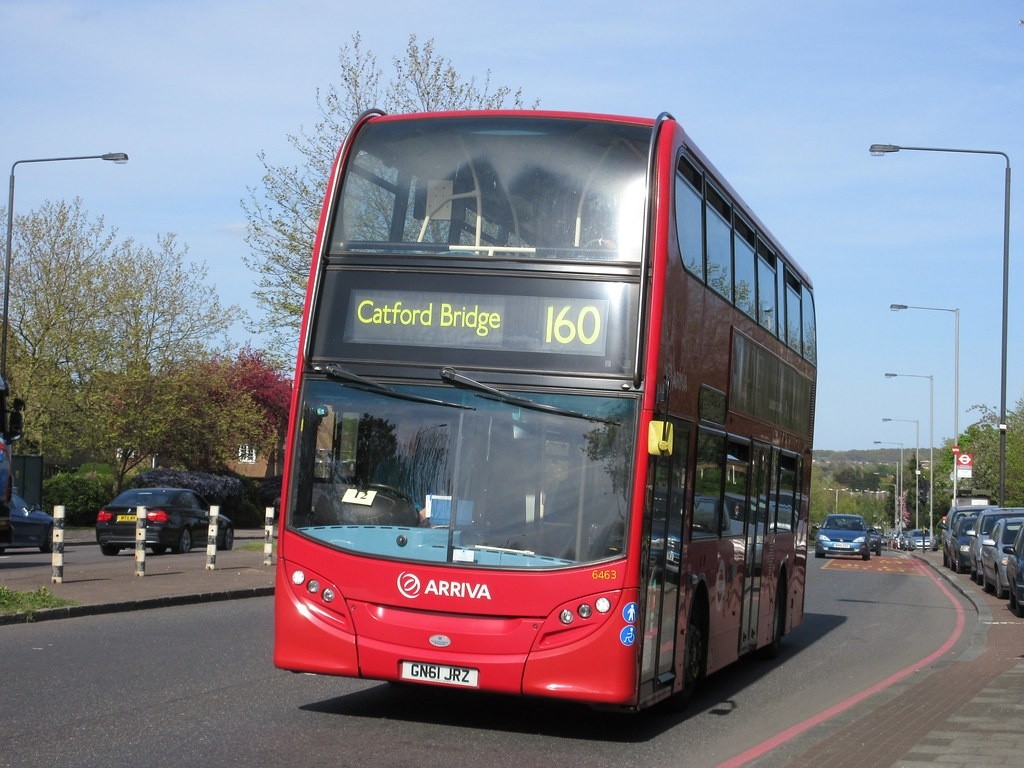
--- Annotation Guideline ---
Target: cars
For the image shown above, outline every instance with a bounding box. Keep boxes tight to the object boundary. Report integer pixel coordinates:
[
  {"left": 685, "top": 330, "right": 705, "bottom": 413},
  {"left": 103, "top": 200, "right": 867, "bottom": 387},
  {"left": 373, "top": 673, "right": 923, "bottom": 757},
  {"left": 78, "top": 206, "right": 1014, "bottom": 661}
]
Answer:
[
  {"left": 95, "top": 488, "right": 234, "bottom": 556},
  {"left": 965, "top": 508, "right": 1024, "bottom": 586},
  {"left": 0, "top": 494, "right": 55, "bottom": 555},
  {"left": 979, "top": 516, "right": 1024, "bottom": 597},
  {"left": 865, "top": 525, "right": 883, "bottom": 556},
  {"left": 948, "top": 514, "right": 979, "bottom": 574},
  {"left": 812, "top": 514, "right": 875, "bottom": 560},
  {"left": 1004, "top": 523, "right": 1024, "bottom": 616},
  {"left": 884, "top": 529, "right": 937, "bottom": 556}
]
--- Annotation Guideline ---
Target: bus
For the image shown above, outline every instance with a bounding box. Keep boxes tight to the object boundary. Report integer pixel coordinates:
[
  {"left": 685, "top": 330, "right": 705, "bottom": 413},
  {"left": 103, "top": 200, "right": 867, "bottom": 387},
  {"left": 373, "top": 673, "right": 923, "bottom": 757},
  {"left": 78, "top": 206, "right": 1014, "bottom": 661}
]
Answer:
[{"left": 270, "top": 109, "right": 818, "bottom": 719}]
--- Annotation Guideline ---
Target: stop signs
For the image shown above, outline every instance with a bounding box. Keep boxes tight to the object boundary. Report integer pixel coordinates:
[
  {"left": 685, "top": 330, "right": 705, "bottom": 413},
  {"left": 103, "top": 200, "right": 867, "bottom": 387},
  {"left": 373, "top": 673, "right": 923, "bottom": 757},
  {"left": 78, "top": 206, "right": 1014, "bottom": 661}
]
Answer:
[{"left": 952, "top": 445, "right": 960, "bottom": 455}]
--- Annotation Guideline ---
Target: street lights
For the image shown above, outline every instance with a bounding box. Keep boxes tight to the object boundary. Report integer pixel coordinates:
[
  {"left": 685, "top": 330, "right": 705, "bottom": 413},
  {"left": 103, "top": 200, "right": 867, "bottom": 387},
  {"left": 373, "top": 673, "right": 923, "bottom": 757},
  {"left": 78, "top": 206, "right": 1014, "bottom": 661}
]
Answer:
[
  {"left": 881, "top": 417, "right": 920, "bottom": 531},
  {"left": 0, "top": 153, "right": 130, "bottom": 387},
  {"left": 874, "top": 441, "right": 903, "bottom": 535},
  {"left": 878, "top": 458, "right": 899, "bottom": 530},
  {"left": 869, "top": 139, "right": 1012, "bottom": 508},
  {"left": 889, "top": 303, "right": 959, "bottom": 509},
  {"left": 885, "top": 373, "right": 934, "bottom": 551}
]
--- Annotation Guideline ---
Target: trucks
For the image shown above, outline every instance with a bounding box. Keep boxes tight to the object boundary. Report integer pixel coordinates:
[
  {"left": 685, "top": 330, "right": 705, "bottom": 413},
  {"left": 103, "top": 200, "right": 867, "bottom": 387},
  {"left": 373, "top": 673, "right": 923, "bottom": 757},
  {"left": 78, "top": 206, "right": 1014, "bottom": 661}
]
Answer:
[{"left": 0, "top": 375, "right": 28, "bottom": 558}]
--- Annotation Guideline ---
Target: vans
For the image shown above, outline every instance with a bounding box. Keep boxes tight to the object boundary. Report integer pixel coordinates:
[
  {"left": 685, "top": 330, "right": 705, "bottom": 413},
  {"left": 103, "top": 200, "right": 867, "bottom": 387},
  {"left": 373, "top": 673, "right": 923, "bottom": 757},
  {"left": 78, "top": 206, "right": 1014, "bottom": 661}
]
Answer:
[{"left": 938, "top": 505, "right": 1000, "bottom": 566}]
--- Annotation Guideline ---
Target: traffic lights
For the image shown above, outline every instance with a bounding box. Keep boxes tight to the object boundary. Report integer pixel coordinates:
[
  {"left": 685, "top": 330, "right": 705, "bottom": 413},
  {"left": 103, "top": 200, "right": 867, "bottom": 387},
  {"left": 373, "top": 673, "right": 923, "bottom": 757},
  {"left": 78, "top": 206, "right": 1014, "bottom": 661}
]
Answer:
[{"left": 919, "top": 479, "right": 927, "bottom": 504}]
